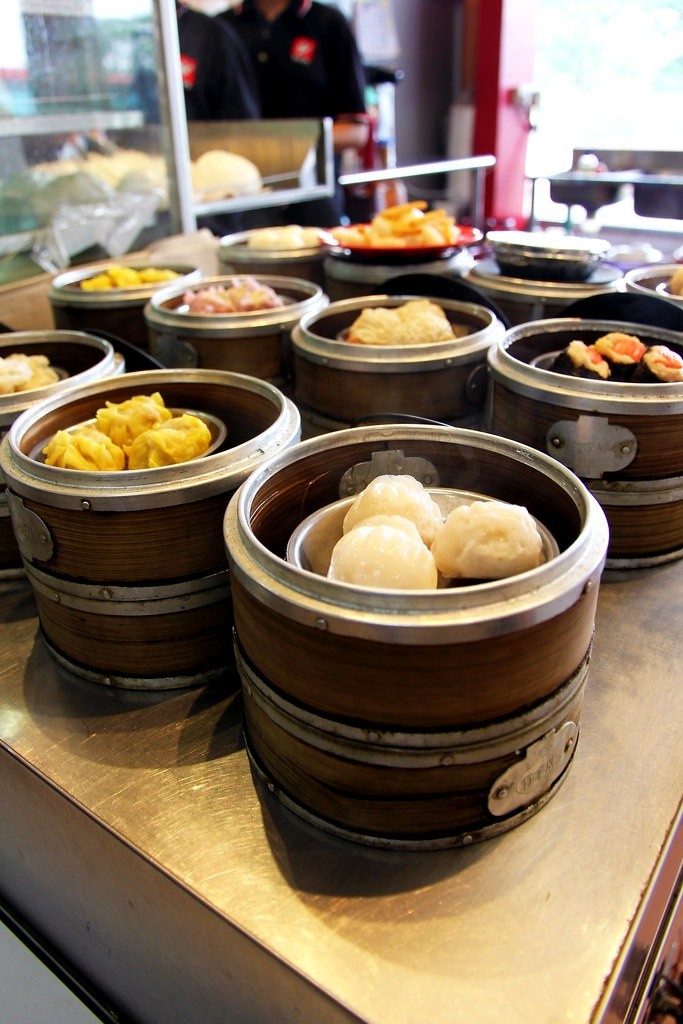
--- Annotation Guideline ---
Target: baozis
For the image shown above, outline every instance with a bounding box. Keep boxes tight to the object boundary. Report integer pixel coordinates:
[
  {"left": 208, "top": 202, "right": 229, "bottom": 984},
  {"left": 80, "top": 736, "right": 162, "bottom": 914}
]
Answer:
[{"left": 41, "top": 391, "right": 211, "bottom": 471}]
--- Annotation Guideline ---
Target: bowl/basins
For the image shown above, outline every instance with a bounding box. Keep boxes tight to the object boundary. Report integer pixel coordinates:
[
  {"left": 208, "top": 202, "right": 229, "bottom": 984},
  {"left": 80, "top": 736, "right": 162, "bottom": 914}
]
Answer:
[
  {"left": 487, "top": 231, "right": 610, "bottom": 282},
  {"left": 174, "top": 295, "right": 296, "bottom": 312},
  {"left": 530, "top": 350, "right": 564, "bottom": 370},
  {"left": 28, "top": 409, "right": 227, "bottom": 465},
  {"left": 655, "top": 281, "right": 681, "bottom": 297},
  {"left": 320, "top": 223, "right": 484, "bottom": 263},
  {"left": 288, "top": 487, "right": 559, "bottom": 577}
]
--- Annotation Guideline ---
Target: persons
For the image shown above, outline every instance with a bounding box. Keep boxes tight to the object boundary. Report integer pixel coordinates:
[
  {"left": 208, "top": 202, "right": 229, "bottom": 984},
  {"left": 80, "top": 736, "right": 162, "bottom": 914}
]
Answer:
[
  {"left": 209, "top": 0, "right": 371, "bottom": 124},
  {"left": 173, "top": 0, "right": 260, "bottom": 125}
]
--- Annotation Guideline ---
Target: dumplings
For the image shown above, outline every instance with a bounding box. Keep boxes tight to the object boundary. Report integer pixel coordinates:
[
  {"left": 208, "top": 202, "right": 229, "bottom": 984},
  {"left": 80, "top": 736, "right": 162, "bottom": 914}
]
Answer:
[{"left": 325, "top": 474, "right": 542, "bottom": 590}]
